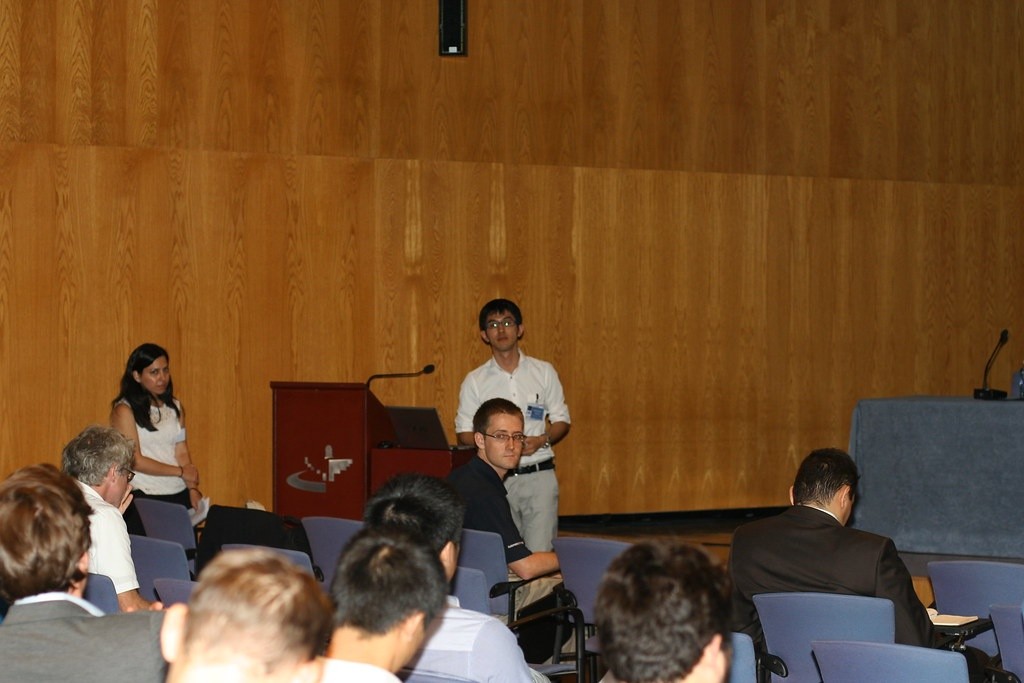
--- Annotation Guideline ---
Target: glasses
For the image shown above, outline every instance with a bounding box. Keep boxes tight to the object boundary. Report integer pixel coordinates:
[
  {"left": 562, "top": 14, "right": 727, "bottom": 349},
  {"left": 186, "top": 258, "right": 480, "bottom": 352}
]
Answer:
[
  {"left": 118, "top": 467, "right": 135, "bottom": 482},
  {"left": 482, "top": 432, "right": 527, "bottom": 443},
  {"left": 484, "top": 319, "right": 518, "bottom": 328}
]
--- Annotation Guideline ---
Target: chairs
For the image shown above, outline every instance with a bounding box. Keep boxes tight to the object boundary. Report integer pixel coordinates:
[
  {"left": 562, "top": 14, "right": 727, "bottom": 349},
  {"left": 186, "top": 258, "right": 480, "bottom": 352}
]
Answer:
[{"left": 82, "top": 498, "right": 1024, "bottom": 683}]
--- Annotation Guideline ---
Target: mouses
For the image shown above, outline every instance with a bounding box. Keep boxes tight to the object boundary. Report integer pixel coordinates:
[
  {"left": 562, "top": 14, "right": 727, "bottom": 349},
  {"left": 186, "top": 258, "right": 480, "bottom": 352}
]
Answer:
[{"left": 380, "top": 441, "right": 394, "bottom": 449}]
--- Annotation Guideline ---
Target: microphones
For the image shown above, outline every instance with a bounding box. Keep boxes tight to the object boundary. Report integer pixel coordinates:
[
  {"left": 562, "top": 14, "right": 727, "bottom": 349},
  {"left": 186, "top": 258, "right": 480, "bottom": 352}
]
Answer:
[
  {"left": 974, "top": 330, "right": 1008, "bottom": 400},
  {"left": 368, "top": 364, "right": 435, "bottom": 387}
]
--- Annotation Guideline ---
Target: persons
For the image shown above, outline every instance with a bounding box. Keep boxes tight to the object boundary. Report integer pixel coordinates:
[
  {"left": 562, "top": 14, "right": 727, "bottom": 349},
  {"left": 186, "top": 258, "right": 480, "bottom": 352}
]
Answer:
[
  {"left": 0, "top": 460, "right": 172, "bottom": 683},
  {"left": 591, "top": 539, "right": 733, "bottom": 683},
  {"left": 723, "top": 446, "right": 994, "bottom": 683},
  {"left": 60, "top": 424, "right": 163, "bottom": 615},
  {"left": 442, "top": 398, "right": 563, "bottom": 620},
  {"left": 455, "top": 299, "right": 571, "bottom": 554},
  {"left": 156, "top": 544, "right": 334, "bottom": 683},
  {"left": 361, "top": 473, "right": 552, "bottom": 683},
  {"left": 311, "top": 522, "right": 447, "bottom": 683},
  {"left": 107, "top": 342, "right": 202, "bottom": 545}
]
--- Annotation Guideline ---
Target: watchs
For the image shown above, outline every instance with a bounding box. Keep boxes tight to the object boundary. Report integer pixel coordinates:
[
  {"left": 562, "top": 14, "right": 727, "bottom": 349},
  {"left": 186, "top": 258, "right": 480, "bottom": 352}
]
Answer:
[{"left": 541, "top": 434, "right": 550, "bottom": 449}]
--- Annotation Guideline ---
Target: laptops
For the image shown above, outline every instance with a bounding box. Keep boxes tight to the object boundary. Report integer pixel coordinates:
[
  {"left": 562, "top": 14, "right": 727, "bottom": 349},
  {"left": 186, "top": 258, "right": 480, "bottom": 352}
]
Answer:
[{"left": 386, "top": 406, "right": 474, "bottom": 450}]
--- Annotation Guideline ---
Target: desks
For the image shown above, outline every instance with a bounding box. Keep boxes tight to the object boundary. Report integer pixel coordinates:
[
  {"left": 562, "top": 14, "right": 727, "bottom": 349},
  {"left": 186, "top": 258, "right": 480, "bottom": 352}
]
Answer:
[{"left": 848, "top": 395, "right": 1024, "bottom": 558}]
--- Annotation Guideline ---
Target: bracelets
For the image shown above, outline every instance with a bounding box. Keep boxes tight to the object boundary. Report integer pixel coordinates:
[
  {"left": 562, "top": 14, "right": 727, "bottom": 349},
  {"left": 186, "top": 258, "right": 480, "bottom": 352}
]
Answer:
[
  {"left": 179, "top": 465, "right": 184, "bottom": 477},
  {"left": 189, "top": 488, "right": 202, "bottom": 498}
]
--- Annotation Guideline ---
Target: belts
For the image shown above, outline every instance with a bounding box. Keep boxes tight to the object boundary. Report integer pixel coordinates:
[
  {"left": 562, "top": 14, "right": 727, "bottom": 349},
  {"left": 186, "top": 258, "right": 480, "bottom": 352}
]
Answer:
[{"left": 508, "top": 459, "right": 555, "bottom": 476}]
